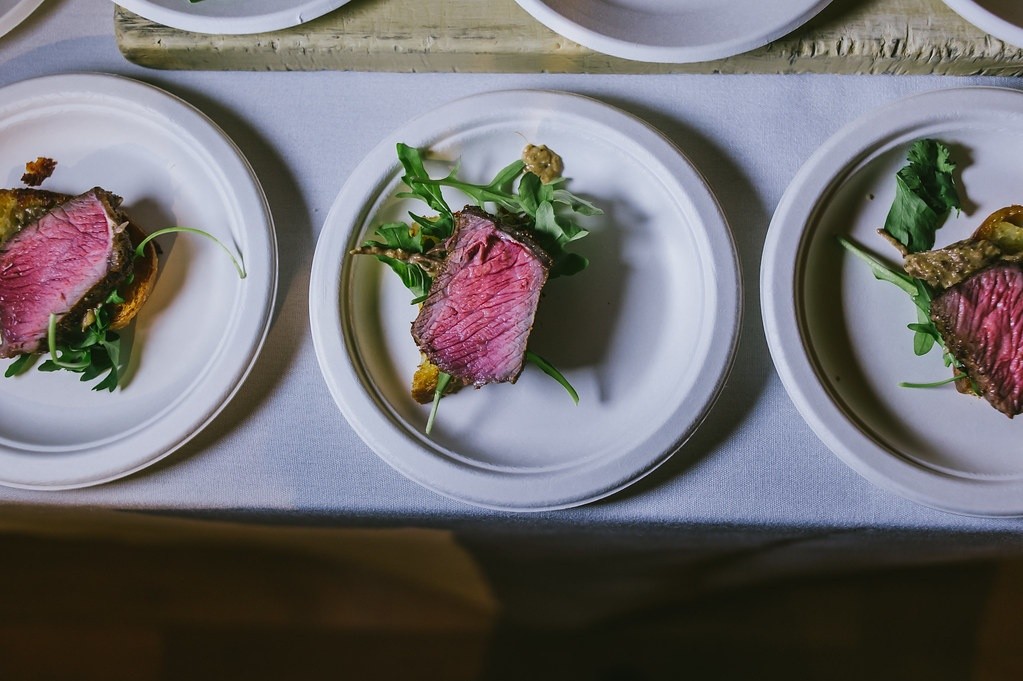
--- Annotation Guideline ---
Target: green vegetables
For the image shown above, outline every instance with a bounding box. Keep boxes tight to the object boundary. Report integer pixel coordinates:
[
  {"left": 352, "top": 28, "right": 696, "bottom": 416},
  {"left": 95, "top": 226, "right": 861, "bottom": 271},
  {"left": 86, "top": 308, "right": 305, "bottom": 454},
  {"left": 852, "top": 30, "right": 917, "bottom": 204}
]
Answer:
[
  {"left": 835, "top": 137, "right": 982, "bottom": 398},
  {"left": 350, "top": 142, "right": 605, "bottom": 436},
  {"left": 4, "top": 227, "right": 245, "bottom": 393}
]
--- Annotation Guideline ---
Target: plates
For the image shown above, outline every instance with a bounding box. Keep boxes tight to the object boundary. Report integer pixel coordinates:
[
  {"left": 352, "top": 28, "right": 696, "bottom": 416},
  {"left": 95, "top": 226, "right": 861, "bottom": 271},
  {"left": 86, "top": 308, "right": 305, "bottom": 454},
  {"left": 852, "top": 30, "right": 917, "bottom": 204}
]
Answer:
[
  {"left": 111, "top": 0, "right": 351, "bottom": 35},
  {"left": 760, "top": 85, "right": 1023, "bottom": 521},
  {"left": 513, "top": 0, "right": 836, "bottom": 63},
  {"left": 0, "top": 74, "right": 278, "bottom": 492},
  {"left": 0, "top": 0, "right": 46, "bottom": 38},
  {"left": 310, "top": 89, "right": 744, "bottom": 512},
  {"left": 940, "top": 0, "right": 1023, "bottom": 49}
]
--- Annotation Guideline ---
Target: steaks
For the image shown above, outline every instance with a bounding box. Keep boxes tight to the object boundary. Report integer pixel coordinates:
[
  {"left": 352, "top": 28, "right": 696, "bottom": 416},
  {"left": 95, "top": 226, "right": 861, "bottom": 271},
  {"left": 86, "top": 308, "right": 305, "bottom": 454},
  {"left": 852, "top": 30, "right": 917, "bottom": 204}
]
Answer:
[
  {"left": 0, "top": 186, "right": 138, "bottom": 359},
  {"left": 927, "top": 260, "right": 1023, "bottom": 420},
  {"left": 410, "top": 203, "right": 555, "bottom": 388}
]
